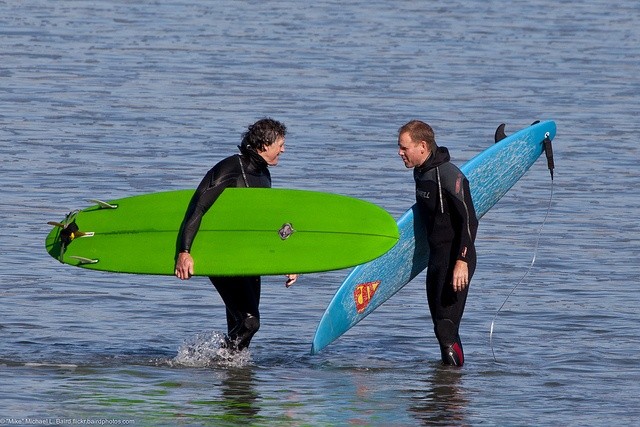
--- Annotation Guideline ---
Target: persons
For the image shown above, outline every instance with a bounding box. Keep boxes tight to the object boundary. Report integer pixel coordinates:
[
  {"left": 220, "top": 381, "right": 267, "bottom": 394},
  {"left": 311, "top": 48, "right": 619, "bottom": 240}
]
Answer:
[
  {"left": 174, "top": 117, "right": 287, "bottom": 350},
  {"left": 398, "top": 120, "right": 478, "bottom": 367}
]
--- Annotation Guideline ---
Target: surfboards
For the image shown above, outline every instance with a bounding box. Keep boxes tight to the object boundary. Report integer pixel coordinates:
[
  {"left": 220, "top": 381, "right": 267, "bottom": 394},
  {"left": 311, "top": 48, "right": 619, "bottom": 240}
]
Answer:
[
  {"left": 311, "top": 120, "right": 557, "bottom": 353},
  {"left": 46, "top": 187, "right": 401, "bottom": 276}
]
